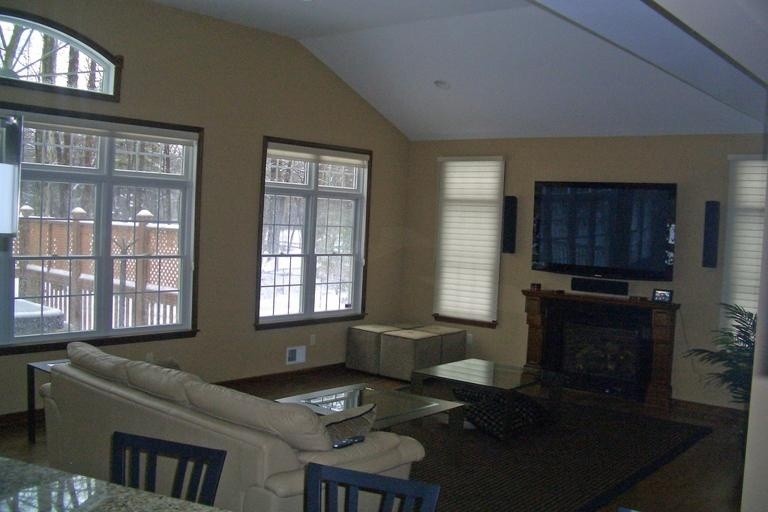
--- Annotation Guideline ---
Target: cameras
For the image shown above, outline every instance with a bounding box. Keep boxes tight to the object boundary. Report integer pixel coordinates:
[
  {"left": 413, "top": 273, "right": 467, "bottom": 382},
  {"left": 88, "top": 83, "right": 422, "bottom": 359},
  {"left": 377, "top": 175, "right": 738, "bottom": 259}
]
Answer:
[{"left": 652, "top": 289, "right": 673, "bottom": 304}]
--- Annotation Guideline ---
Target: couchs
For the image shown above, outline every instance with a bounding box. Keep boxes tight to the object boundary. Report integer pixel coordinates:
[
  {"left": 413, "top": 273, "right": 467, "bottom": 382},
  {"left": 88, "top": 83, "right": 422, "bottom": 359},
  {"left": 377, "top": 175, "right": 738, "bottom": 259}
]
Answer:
[
  {"left": 343, "top": 320, "right": 467, "bottom": 386},
  {"left": 38, "top": 339, "right": 427, "bottom": 512}
]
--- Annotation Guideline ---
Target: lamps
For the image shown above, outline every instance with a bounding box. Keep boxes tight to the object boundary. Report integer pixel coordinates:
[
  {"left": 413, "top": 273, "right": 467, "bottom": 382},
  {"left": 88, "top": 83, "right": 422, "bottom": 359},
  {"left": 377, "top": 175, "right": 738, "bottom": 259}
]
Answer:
[{"left": 1, "top": 115, "right": 22, "bottom": 247}]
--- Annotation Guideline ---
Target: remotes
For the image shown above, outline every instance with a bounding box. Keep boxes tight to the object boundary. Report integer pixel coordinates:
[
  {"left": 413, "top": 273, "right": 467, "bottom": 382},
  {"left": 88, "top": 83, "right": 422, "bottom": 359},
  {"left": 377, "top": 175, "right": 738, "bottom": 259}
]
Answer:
[{"left": 333, "top": 436, "right": 365, "bottom": 449}]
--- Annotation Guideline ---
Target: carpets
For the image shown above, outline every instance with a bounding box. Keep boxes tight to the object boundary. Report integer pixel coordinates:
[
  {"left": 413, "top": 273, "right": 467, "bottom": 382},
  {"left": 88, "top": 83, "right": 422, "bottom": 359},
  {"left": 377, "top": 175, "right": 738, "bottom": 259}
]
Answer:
[{"left": 380, "top": 399, "right": 713, "bottom": 511}]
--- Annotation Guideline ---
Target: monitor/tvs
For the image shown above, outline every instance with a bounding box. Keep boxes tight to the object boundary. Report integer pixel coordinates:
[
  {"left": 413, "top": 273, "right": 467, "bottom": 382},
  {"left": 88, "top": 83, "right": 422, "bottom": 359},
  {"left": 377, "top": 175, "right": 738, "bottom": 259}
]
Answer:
[{"left": 531, "top": 181, "right": 677, "bottom": 282}]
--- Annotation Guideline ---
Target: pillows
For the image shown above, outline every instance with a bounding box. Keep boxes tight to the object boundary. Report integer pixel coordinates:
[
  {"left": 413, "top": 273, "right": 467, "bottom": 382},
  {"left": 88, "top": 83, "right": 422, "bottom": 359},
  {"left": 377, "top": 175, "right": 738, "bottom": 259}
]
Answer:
[{"left": 462, "top": 384, "right": 558, "bottom": 443}]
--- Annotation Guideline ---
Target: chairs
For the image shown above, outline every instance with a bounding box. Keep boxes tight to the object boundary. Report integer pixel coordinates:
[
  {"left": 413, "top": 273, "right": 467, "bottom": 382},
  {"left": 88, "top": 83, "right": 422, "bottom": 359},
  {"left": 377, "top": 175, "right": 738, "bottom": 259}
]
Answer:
[
  {"left": 110, "top": 428, "right": 228, "bottom": 505},
  {"left": 302, "top": 459, "right": 443, "bottom": 511}
]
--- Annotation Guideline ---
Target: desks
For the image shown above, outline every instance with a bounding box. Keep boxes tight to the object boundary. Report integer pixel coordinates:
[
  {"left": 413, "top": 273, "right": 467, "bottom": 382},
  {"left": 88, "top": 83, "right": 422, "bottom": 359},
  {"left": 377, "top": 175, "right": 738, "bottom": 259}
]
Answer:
[
  {"left": 23, "top": 356, "right": 73, "bottom": 444},
  {"left": 273, "top": 382, "right": 466, "bottom": 435},
  {"left": 412, "top": 356, "right": 542, "bottom": 392},
  {"left": 0, "top": 453, "right": 230, "bottom": 512}
]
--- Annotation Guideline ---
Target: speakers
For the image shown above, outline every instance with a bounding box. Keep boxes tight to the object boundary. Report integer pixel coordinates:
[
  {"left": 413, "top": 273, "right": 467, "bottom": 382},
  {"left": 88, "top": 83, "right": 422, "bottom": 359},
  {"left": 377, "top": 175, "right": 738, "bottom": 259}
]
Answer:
[
  {"left": 571, "top": 278, "right": 628, "bottom": 295},
  {"left": 502, "top": 196, "right": 517, "bottom": 253},
  {"left": 702, "top": 201, "right": 720, "bottom": 267}
]
